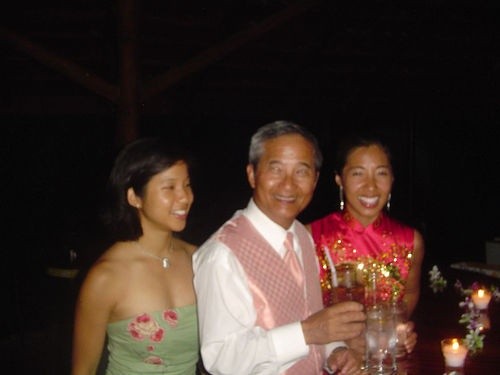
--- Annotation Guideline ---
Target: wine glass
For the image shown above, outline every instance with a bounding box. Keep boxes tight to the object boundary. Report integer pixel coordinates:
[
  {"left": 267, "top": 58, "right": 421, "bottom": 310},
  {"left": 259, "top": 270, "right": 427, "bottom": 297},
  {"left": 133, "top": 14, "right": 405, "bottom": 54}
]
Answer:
[{"left": 367, "top": 312, "right": 397, "bottom": 375}]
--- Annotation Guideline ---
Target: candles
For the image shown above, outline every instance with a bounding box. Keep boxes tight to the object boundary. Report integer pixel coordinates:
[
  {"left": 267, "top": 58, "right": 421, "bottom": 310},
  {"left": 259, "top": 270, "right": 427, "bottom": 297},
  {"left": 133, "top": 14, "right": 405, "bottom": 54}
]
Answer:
[
  {"left": 442, "top": 339, "right": 469, "bottom": 366},
  {"left": 472, "top": 289, "right": 491, "bottom": 309}
]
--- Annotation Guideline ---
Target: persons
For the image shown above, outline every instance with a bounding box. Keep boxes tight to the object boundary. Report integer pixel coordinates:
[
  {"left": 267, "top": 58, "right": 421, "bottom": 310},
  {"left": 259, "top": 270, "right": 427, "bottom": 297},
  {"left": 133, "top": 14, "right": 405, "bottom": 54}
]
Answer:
[
  {"left": 191, "top": 121, "right": 368, "bottom": 375},
  {"left": 302, "top": 128, "right": 425, "bottom": 375},
  {"left": 71, "top": 137, "right": 206, "bottom": 375}
]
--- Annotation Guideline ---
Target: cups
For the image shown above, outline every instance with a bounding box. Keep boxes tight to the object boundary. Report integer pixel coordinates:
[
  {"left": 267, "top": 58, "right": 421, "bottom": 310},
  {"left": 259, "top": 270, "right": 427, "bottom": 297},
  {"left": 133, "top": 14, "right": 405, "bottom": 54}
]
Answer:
[
  {"left": 441, "top": 339, "right": 469, "bottom": 371},
  {"left": 329, "top": 271, "right": 356, "bottom": 302}
]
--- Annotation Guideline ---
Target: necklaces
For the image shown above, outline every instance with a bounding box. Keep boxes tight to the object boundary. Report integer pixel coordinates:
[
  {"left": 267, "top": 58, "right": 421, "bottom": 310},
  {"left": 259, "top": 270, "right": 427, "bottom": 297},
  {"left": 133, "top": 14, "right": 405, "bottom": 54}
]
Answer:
[{"left": 135, "top": 233, "right": 174, "bottom": 268}]
